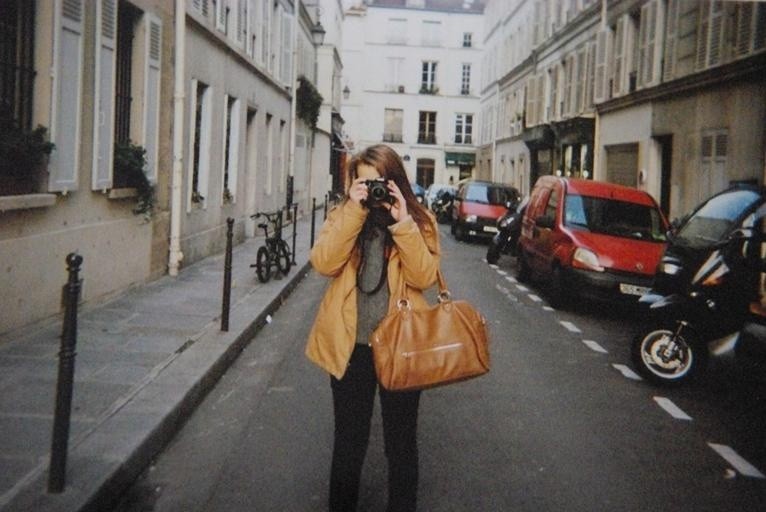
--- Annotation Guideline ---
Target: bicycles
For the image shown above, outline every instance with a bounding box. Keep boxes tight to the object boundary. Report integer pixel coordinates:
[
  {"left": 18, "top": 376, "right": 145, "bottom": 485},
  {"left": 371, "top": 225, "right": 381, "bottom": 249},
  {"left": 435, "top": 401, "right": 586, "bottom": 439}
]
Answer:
[{"left": 250, "top": 207, "right": 294, "bottom": 283}]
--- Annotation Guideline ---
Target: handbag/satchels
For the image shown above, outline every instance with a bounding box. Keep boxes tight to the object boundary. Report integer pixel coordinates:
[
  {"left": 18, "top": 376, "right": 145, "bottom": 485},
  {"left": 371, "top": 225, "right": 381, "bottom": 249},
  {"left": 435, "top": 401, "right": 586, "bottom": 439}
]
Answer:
[{"left": 367, "top": 296, "right": 493, "bottom": 394}]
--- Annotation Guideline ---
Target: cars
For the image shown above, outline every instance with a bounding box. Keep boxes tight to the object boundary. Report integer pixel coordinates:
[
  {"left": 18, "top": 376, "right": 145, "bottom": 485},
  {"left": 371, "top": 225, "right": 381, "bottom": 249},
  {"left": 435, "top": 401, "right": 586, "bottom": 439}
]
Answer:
[
  {"left": 452, "top": 180, "right": 517, "bottom": 243},
  {"left": 514, "top": 173, "right": 674, "bottom": 308}
]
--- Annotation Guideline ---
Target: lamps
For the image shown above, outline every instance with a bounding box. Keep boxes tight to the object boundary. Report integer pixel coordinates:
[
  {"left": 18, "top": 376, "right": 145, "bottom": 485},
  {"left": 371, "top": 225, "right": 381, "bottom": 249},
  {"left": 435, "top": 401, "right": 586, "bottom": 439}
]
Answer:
[
  {"left": 343, "top": 85, "right": 350, "bottom": 99},
  {"left": 305, "top": 3, "right": 326, "bottom": 46}
]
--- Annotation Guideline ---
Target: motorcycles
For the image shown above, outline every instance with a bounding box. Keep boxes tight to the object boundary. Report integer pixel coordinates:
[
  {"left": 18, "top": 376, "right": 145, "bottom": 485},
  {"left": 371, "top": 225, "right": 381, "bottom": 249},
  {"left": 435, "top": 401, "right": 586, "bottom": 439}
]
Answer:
[
  {"left": 485, "top": 199, "right": 520, "bottom": 264},
  {"left": 630, "top": 227, "right": 764, "bottom": 387}
]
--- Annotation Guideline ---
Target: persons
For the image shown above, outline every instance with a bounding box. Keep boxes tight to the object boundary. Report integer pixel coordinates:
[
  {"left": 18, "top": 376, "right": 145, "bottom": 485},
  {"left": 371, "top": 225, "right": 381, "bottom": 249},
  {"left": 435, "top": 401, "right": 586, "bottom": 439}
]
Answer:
[
  {"left": 441, "top": 191, "right": 455, "bottom": 225},
  {"left": 304, "top": 144, "right": 440, "bottom": 512}
]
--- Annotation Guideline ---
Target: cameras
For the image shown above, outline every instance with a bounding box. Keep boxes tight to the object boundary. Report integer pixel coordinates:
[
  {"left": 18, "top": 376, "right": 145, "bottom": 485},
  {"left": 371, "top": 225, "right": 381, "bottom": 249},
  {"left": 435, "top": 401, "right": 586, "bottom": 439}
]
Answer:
[{"left": 358, "top": 177, "right": 394, "bottom": 206}]
